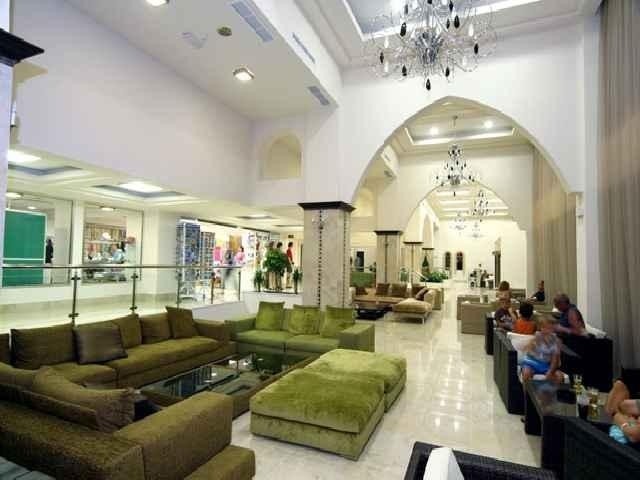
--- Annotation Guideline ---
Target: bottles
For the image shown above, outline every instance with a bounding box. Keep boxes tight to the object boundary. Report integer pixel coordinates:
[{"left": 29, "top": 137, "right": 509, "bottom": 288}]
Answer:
[
  {"left": 191, "top": 372, "right": 195, "bottom": 385},
  {"left": 205, "top": 366, "right": 209, "bottom": 380},
  {"left": 591, "top": 404, "right": 601, "bottom": 422},
  {"left": 195, "top": 373, "right": 199, "bottom": 386},
  {"left": 209, "top": 366, "right": 212, "bottom": 380},
  {"left": 202, "top": 368, "right": 205, "bottom": 378},
  {"left": 199, "top": 371, "right": 203, "bottom": 385},
  {"left": 591, "top": 390, "right": 599, "bottom": 404}
]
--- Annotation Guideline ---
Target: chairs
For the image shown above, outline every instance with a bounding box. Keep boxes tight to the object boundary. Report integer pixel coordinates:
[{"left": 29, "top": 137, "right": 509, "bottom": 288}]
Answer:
[
  {"left": 402, "top": 441, "right": 556, "bottom": 479},
  {"left": 564, "top": 415, "right": 640, "bottom": 480},
  {"left": 500, "top": 338, "right": 586, "bottom": 416},
  {"left": 620, "top": 366, "right": 640, "bottom": 393}
]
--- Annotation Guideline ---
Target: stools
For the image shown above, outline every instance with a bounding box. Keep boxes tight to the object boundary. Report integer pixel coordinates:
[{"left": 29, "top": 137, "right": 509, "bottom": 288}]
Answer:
[{"left": 248, "top": 348, "right": 409, "bottom": 463}]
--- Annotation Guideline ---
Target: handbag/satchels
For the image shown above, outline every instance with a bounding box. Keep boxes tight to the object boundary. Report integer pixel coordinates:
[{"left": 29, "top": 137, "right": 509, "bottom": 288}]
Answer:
[{"left": 253, "top": 354, "right": 281, "bottom": 377}]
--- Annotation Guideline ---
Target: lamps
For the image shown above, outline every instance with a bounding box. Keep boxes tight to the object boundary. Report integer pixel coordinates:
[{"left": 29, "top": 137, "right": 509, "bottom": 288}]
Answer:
[
  {"left": 448, "top": 212, "right": 468, "bottom": 234},
  {"left": 359, "top": 0, "right": 499, "bottom": 90},
  {"left": 468, "top": 188, "right": 494, "bottom": 223},
  {"left": 145, "top": 0, "right": 167, "bottom": 8},
  {"left": 232, "top": 68, "right": 254, "bottom": 84},
  {"left": 469, "top": 222, "right": 485, "bottom": 239}
]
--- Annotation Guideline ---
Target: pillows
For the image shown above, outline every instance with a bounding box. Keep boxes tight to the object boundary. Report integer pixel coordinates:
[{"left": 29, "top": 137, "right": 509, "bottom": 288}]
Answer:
[
  {"left": 0, "top": 307, "right": 197, "bottom": 434},
  {"left": 355, "top": 283, "right": 428, "bottom": 301},
  {"left": 255, "top": 302, "right": 353, "bottom": 339}
]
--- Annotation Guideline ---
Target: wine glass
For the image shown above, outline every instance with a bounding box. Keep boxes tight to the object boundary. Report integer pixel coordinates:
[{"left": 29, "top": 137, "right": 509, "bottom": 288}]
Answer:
[{"left": 575, "top": 374, "right": 582, "bottom": 391}]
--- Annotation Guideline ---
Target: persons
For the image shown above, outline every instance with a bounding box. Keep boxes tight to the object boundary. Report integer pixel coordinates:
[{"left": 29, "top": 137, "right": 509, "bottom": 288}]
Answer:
[
  {"left": 108, "top": 244, "right": 125, "bottom": 280},
  {"left": 46, "top": 240, "right": 53, "bottom": 263},
  {"left": 234, "top": 246, "right": 246, "bottom": 264},
  {"left": 212, "top": 246, "right": 220, "bottom": 275},
  {"left": 470, "top": 263, "right": 639, "bottom": 445},
  {"left": 275, "top": 243, "right": 283, "bottom": 290},
  {"left": 286, "top": 242, "right": 294, "bottom": 288},
  {"left": 88, "top": 244, "right": 99, "bottom": 278}
]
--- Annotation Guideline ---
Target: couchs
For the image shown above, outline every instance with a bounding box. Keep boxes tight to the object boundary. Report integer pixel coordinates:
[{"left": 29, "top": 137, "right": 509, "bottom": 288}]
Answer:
[
  {"left": 492, "top": 327, "right": 614, "bottom": 390},
  {"left": 349, "top": 286, "right": 444, "bottom": 324},
  {"left": 427, "top": 112, "right": 479, "bottom": 198},
  {"left": 224, "top": 314, "right": 375, "bottom": 358},
  {"left": 456, "top": 288, "right": 553, "bottom": 355}
]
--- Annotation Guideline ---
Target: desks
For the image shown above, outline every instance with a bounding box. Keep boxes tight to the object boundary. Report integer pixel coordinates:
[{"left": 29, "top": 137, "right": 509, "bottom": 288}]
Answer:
[{"left": 523, "top": 379, "right": 615, "bottom": 473}]
